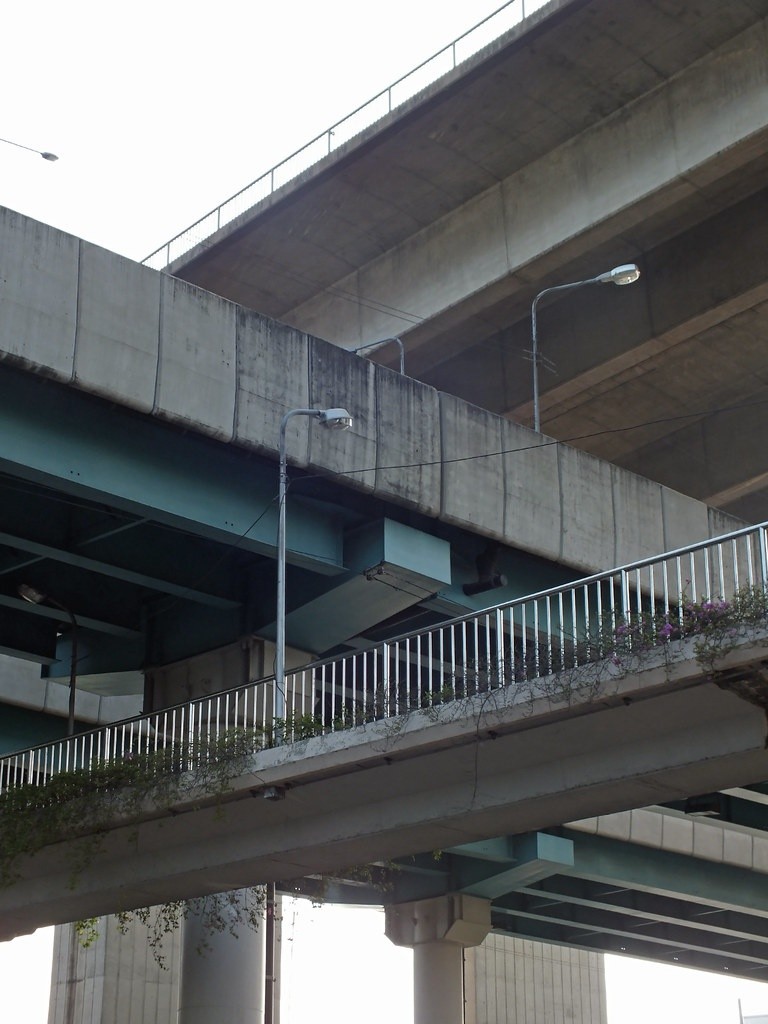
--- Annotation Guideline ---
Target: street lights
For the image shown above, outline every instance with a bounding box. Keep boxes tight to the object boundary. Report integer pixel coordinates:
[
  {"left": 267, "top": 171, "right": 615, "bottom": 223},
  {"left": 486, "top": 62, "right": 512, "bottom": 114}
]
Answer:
[
  {"left": 349, "top": 337, "right": 404, "bottom": 375},
  {"left": 18, "top": 582, "right": 78, "bottom": 738},
  {"left": 531, "top": 263, "right": 640, "bottom": 433},
  {"left": 273, "top": 408, "right": 354, "bottom": 745}
]
